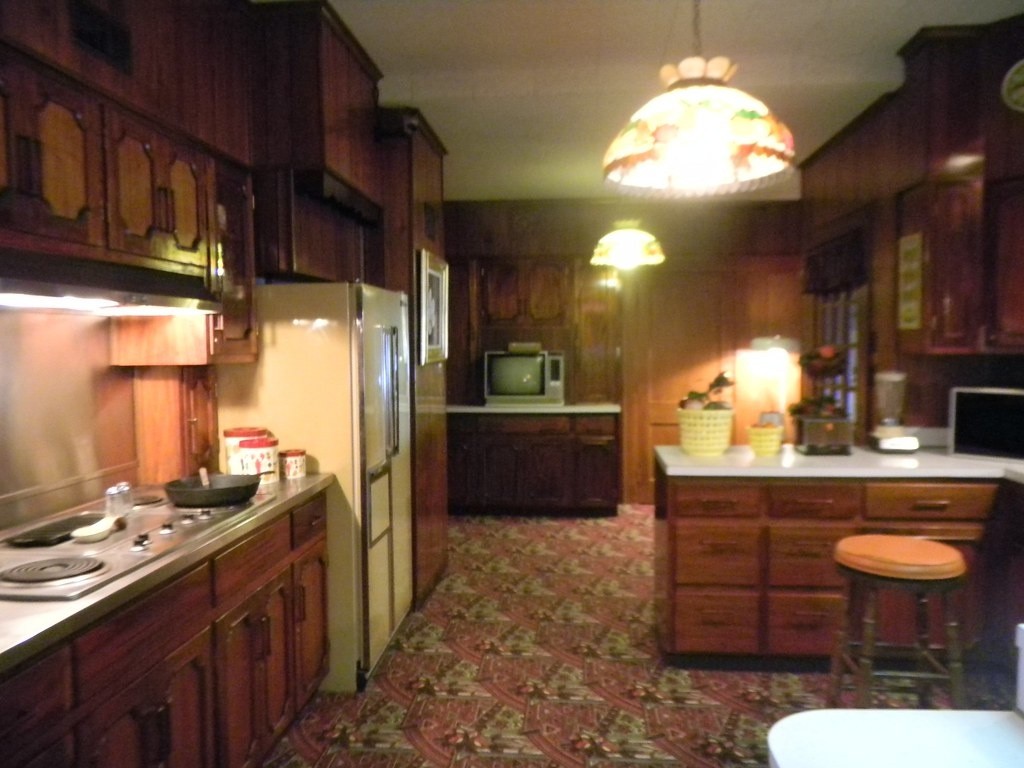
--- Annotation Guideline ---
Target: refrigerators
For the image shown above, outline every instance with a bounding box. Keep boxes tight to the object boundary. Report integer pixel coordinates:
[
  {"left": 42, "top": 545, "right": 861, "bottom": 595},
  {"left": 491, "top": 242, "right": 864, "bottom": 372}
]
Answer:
[{"left": 213, "top": 280, "right": 414, "bottom": 695}]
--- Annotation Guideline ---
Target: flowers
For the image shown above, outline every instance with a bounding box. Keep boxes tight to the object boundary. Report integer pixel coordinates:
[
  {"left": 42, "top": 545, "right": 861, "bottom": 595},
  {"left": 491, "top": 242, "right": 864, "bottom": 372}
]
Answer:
[
  {"left": 797, "top": 340, "right": 847, "bottom": 366},
  {"left": 789, "top": 396, "right": 848, "bottom": 419}
]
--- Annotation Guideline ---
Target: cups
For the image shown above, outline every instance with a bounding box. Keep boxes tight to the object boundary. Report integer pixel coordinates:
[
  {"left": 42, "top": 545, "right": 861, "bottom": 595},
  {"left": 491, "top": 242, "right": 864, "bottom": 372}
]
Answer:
[{"left": 873, "top": 371, "right": 908, "bottom": 426}]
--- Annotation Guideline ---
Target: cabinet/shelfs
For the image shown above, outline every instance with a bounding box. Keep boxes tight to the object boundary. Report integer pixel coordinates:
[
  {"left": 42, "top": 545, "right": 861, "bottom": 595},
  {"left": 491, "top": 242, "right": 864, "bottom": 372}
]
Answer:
[
  {"left": 72, "top": 562, "right": 218, "bottom": 768},
  {"left": 133, "top": 365, "right": 220, "bottom": 485},
  {"left": 211, "top": 511, "right": 297, "bottom": 768},
  {"left": 653, "top": 459, "right": 1002, "bottom": 670},
  {"left": 0, "top": 639, "right": 76, "bottom": 768},
  {"left": 292, "top": 490, "right": 331, "bottom": 717},
  {"left": 0, "top": 0, "right": 258, "bottom": 171},
  {"left": 250, "top": 0, "right": 384, "bottom": 280},
  {"left": 101, "top": 99, "right": 208, "bottom": 277},
  {"left": 802, "top": 13, "right": 1024, "bottom": 440},
  {"left": 110, "top": 165, "right": 262, "bottom": 365},
  {"left": 446, "top": 413, "right": 623, "bottom": 515},
  {"left": 0, "top": 44, "right": 108, "bottom": 266}
]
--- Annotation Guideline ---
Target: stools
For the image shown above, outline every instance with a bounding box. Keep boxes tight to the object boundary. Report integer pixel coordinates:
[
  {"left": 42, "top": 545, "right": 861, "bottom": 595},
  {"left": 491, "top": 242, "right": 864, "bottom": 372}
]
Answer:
[{"left": 825, "top": 534, "right": 968, "bottom": 708}]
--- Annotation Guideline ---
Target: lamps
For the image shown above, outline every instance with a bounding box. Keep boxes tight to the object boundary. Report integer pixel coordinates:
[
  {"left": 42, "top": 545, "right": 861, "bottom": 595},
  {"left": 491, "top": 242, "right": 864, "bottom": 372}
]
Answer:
[
  {"left": 601, "top": 0, "right": 796, "bottom": 198},
  {"left": 588, "top": 204, "right": 666, "bottom": 271}
]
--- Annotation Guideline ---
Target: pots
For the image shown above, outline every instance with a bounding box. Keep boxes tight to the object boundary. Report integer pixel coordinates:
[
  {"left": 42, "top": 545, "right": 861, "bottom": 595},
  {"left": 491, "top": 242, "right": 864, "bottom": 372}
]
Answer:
[{"left": 162, "top": 470, "right": 276, "bottom": 507}]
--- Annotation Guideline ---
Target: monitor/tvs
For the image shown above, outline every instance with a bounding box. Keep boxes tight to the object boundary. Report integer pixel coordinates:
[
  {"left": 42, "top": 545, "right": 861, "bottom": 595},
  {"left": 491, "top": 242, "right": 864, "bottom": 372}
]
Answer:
[{"left": 484, "top": 352, "right": 564, "bottom": 407}]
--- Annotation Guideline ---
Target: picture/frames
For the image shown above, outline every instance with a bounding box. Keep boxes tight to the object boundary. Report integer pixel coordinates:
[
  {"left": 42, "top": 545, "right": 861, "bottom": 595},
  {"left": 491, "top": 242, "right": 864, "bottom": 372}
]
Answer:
[{"left": 419, "top": 248, "right": 449, "bottom": 367}]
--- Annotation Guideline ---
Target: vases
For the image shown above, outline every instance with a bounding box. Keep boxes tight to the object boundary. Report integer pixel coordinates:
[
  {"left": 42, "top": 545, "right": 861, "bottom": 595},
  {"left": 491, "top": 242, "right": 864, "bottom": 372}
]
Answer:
[
  {"left": 802, "top": 354, "right": 846, "bottom": 378},
  {"left": 794, "top": 416, "right": 853, "bottom": 457}
]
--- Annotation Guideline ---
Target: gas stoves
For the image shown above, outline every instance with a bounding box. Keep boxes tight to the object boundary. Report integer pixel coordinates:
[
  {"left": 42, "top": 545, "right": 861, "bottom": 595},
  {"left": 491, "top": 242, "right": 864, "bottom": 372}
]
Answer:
[{"left": 0, "top": 489, "right": 276, "bottom": 600}]
